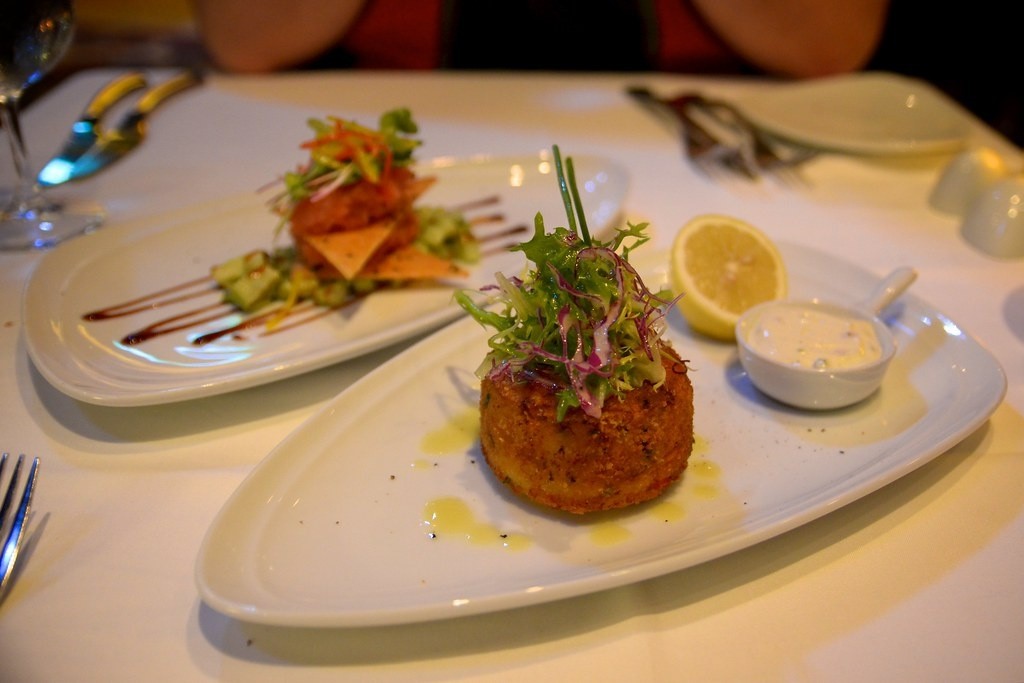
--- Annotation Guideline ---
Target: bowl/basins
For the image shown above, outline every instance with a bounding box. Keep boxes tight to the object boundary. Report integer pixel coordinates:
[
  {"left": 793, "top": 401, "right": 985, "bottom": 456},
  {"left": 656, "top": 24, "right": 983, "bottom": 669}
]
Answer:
[{"left": 736, "top": 297, "right": 898, "bottom": 412}]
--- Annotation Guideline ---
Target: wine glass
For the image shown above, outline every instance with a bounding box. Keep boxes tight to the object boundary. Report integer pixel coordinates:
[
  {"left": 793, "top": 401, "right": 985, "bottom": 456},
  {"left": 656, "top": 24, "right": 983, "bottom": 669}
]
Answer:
[{"left": 0, "top": 0, "right": 76, "bottom": 253}]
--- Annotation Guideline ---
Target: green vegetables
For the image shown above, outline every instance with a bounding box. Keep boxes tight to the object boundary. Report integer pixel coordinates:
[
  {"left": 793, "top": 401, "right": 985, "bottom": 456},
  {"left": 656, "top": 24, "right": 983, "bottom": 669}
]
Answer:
[
  {"left": 453, "top": 145, "right": 686, "bottom": 423},
  {"left": 279, "top": 106, "right": 426, "bottom": 211}
]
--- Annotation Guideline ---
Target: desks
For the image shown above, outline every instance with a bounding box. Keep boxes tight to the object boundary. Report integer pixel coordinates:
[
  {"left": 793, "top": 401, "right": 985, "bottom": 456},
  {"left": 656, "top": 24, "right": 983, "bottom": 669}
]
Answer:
[{"left": 0, "top": 72, "right": 1023, "bottom": 683}]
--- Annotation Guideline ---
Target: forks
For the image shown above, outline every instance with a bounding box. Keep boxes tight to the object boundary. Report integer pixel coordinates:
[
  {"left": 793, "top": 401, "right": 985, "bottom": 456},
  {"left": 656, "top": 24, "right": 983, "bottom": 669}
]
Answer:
[
  {"left": 0, "top": 451, "right": 41, "bottom": 597},
  {"left": 625, "top": 84, "right": 821, "bottom": 184}
]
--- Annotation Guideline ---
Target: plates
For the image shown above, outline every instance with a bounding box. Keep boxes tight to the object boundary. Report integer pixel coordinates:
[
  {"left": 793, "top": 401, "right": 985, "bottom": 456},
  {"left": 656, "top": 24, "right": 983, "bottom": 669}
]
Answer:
[
  {"left": 19, "top": 152, "right": 632, "bottom": 407},
  {"left": 195, "top": 237, "right": 1009, "bottom": 629},
  {"left": 724, "top": 74, "right": 979, "bottom": 166}
]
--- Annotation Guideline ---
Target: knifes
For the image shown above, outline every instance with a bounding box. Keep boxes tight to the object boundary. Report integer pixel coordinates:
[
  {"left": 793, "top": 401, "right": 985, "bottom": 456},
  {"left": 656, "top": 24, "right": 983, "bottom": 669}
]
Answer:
[{"left": 32, "top": 67, "right": 208, "bottom": 190}]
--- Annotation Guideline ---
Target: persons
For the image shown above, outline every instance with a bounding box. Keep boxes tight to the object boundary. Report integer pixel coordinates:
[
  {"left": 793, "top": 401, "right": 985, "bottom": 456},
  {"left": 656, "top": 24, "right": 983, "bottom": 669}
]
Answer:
[{"left": 194, "top": 0, "right": 891, "bottom": 79}]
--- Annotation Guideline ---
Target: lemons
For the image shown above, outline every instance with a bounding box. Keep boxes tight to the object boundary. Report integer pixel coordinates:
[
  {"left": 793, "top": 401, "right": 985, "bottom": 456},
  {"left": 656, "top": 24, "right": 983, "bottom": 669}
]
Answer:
[{"left": 671, "top": 216, "right": 787, "bottom": 338}]
